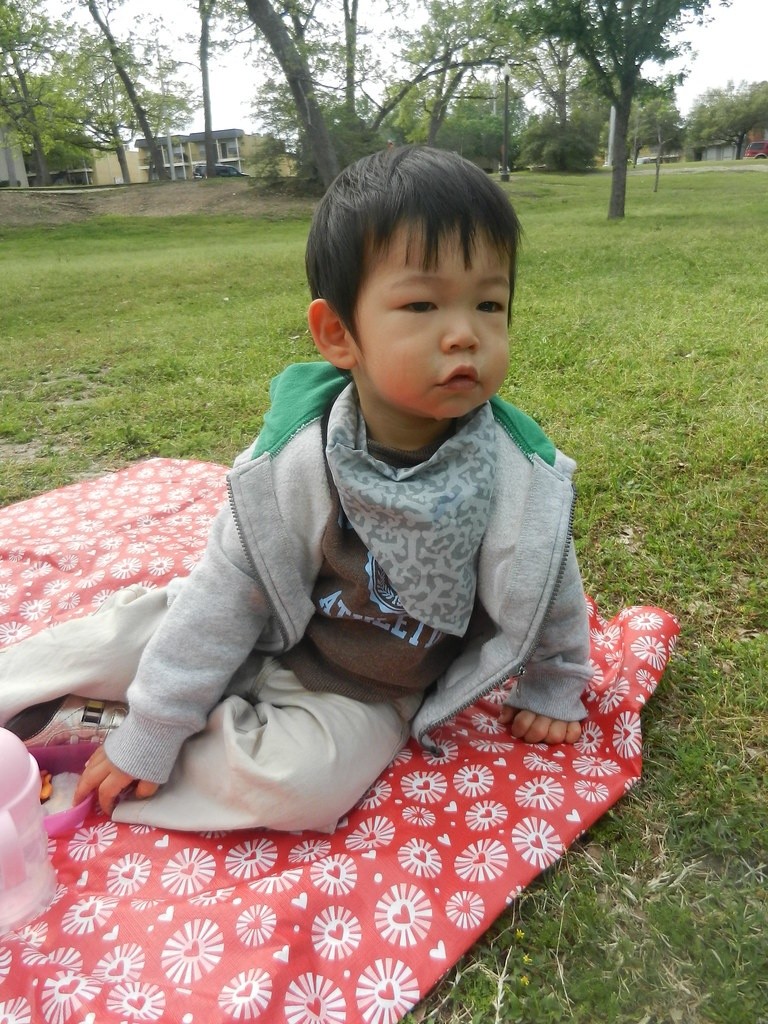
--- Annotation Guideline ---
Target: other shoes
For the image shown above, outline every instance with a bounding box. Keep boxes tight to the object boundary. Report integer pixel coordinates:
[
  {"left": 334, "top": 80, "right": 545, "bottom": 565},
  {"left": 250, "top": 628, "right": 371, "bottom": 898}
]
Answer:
[{"left": 3, "top": 693, "right": 130, "bottom": 749}]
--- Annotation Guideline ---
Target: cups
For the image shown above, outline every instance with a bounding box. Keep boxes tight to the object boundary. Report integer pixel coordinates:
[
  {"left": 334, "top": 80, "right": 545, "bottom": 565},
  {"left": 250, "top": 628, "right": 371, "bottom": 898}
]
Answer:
[{"left": 0, "top": 727, "right": 57, "bottom": 935}]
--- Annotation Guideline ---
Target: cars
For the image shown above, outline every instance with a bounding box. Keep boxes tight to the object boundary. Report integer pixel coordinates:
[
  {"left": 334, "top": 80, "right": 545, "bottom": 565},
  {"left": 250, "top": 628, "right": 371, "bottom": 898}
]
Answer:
[{"left": 636, "top": 157, "right": 652, "bottom": 166}]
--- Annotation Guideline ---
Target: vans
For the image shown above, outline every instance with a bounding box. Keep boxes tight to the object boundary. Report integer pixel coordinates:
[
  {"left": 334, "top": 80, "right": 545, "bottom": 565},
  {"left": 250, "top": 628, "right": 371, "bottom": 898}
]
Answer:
[{"left": 743, "top": 140, "right": 768, "bottom": 162}]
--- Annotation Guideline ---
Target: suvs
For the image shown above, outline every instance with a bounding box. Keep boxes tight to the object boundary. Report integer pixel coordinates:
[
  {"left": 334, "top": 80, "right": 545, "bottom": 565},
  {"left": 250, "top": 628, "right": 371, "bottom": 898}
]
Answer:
[{"left": 192, "top": 163, "right": 250, "bottom": 179}]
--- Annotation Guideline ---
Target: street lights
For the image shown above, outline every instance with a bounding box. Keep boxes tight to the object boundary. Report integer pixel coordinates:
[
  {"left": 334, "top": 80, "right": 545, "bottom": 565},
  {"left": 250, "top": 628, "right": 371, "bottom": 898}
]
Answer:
[{"left": 500, "top": 62, "right": 512, "bottom": 182}]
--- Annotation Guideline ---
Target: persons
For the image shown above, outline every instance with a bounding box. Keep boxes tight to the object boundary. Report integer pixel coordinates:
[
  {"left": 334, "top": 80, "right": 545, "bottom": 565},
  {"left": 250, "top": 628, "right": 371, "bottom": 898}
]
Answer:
[{"left": 4, "top": 144, "right": 592, "bottom": 830}]
